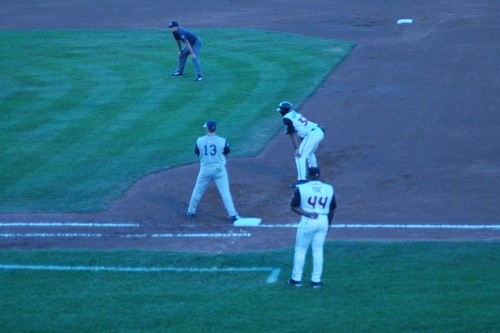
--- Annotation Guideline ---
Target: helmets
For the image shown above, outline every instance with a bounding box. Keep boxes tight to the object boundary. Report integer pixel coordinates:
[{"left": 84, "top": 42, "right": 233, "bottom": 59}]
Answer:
[
  {"left": 276, "top": 101, "right": 292, "bottom": 112},
  {"left": 204, "top": 120, "right": 216, "bottom": 130}
]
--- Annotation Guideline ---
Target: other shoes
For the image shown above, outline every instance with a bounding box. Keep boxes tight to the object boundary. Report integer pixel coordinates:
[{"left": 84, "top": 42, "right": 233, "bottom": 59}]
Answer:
[
  {"left": 229, "top": 215, "right": 240, "bottom": 222},
  {"left": 286, "top": 279, "right": 302, "bottom": 287},
  {"left": 195, "top": 77, "right": 202, "bottom": 81},
  {"left": 289, "top": 180, "right": 305, "bottom": 187},
  {"left": 185, "top": 212, "right": 194, "bottom": 217},
  {"left": 171, "top": 72, "right": 182, "bottom": 76},
  {"left": 313, "top": 282, "right": 321, "bottom": 288}
]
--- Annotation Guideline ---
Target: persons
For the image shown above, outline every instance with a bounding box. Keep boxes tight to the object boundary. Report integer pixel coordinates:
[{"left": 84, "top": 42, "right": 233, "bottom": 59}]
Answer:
[
  {"left": 184, "top": 120, "right": 240, "bottom": 223},
  {"left": 285, "top": 167, "right": 336, "bottom": 289},
  {"left": 277, "top": 101, "right": 325, "bottom": 188},
  {"left": 168, "top": 21, "right": 202, "bottom": 81}
]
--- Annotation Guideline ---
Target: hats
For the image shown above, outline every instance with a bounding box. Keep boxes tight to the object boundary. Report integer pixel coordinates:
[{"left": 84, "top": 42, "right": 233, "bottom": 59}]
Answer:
[
  {"left": 306, "top": 167, "right": 320, "bottom": 176},
  {"left": 168, "top": 21, "right": 178, "bottom": 27}
]
